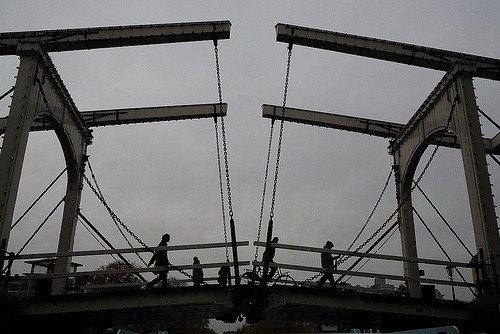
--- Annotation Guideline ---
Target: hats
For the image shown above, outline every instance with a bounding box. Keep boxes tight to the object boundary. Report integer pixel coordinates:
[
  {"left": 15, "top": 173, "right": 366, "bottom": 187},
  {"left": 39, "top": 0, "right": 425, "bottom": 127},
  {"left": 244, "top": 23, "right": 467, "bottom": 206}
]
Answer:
[{"left": 327, "top": 241, "right": 334, "bottom": 248}]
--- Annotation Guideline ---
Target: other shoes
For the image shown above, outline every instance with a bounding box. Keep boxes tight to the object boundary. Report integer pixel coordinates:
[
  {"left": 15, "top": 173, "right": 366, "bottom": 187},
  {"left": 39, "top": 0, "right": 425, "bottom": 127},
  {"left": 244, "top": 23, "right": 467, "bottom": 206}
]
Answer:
[{"left": 141, "top": 286, "right": 146, "bottom": 290}]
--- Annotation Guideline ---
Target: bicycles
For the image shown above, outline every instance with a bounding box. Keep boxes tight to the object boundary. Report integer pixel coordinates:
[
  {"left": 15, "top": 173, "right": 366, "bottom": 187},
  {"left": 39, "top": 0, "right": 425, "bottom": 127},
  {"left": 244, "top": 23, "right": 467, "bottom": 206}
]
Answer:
[{"left": 239, "top": 267, "right": 298, "bottom": 287}]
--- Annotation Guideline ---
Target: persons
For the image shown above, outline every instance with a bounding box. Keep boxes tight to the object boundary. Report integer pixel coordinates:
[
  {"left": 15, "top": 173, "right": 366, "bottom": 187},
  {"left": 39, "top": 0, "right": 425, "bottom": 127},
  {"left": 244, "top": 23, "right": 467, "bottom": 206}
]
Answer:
[
  {"left": 140, "top": 234, "right": 174, "bottom": 289},
  {"left": 261, "top": 237, "right": 278, "bottom": 283},
  {"left": 316, "top": 241, "right": 341, "bottom": 288},
  {"left": 216, "top": 267, "right": 227, "bottom": 286},
  {"left": 192, "top": 256, "right": 203, "bottom": 286}
]
